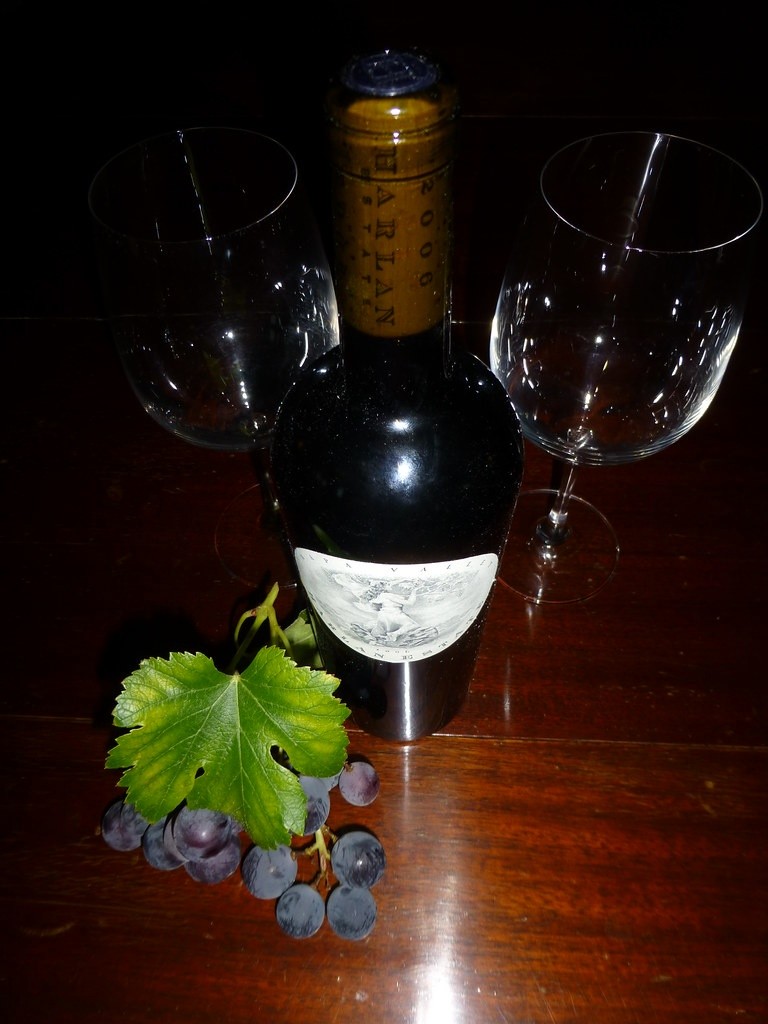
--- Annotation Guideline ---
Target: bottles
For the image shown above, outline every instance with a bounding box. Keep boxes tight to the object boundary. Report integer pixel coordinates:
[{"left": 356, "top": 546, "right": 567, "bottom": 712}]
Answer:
[{"left": 269, "top": 44, "right": 522, "bottom": 739}]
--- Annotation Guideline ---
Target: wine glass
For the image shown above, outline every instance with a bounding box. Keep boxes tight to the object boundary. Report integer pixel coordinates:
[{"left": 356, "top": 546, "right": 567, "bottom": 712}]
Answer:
[
  {"left": 489, "top": 135, "right": 765, "bottom": 606},
  {"left": 84, "top": 129, "right": 338, "bottom": 590}
]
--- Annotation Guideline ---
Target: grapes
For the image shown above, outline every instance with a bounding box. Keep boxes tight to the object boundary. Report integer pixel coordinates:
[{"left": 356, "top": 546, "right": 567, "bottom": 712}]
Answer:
[{"left": 100, "top": 761, "right": 387, "bottom": 942}]
